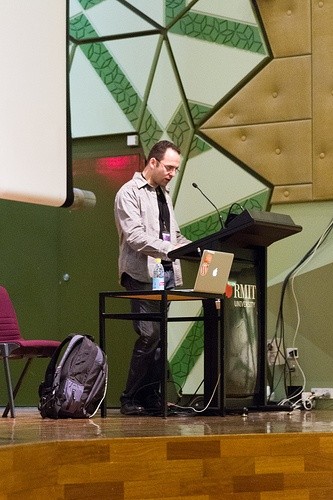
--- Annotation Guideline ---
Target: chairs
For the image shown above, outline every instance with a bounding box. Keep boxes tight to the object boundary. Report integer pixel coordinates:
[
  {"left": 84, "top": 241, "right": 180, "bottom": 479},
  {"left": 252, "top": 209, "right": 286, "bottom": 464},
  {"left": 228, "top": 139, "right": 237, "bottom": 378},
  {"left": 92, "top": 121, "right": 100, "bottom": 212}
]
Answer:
[{"left": 0, "top": 286, "right": 62, "bottom": 419}]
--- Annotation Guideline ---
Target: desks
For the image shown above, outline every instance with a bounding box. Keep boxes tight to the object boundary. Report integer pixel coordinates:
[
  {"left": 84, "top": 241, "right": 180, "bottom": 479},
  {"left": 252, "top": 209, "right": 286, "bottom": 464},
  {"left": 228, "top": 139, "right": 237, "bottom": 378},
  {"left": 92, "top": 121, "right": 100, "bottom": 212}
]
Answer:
[{"left": 99, "top": 291, "right": 221, "bottom": 419}]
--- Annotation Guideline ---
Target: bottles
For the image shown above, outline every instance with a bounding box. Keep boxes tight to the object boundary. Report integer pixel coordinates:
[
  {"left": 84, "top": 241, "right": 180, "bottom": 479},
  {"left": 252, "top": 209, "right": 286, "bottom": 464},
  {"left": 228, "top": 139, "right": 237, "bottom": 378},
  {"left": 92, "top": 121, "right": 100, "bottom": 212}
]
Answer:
[{"left": 152, "top": 258, "right": 165, "bottom": 290}]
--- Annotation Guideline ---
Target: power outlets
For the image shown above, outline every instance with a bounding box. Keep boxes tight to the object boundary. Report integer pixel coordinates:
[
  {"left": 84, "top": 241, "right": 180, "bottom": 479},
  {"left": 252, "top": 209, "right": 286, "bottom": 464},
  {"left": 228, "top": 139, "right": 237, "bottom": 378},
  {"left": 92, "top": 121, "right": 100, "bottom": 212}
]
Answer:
[
  {"left": 312, "top": 388, "right": 333, "bottom": 399},
  {"left": 287, "top": 347, "right": 298, "bottom": 358}
]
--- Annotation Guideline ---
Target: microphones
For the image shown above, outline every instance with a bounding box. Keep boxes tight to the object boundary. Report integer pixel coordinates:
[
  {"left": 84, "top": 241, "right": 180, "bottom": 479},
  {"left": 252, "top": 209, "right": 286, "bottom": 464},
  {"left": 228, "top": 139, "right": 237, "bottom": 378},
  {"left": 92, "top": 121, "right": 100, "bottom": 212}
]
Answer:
[{"left": 192, "top": 183, "right": 223, "bottom": 230}]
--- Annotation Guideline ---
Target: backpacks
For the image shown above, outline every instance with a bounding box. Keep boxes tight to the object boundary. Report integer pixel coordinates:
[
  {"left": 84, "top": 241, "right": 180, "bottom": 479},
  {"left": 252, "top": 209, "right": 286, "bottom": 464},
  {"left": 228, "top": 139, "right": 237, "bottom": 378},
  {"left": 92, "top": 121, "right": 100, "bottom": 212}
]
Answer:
[{"left": 39, "top": 334, "right": 109, "bottom": 418}]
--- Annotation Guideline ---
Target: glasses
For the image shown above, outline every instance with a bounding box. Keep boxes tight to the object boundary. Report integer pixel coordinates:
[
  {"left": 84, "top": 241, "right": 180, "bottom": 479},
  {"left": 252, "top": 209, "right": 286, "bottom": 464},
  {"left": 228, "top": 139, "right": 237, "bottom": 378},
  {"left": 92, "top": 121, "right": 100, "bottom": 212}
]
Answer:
[{"left": 155, "top": 158, "right": 179, "bottom": 172}]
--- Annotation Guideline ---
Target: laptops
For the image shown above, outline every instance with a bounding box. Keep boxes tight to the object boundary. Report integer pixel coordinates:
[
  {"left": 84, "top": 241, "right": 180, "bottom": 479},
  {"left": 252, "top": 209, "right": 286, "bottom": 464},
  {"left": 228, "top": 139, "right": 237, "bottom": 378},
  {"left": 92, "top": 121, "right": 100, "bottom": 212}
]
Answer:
[{"left": 171, "top": 249, "right": 234, "bottom": 294}]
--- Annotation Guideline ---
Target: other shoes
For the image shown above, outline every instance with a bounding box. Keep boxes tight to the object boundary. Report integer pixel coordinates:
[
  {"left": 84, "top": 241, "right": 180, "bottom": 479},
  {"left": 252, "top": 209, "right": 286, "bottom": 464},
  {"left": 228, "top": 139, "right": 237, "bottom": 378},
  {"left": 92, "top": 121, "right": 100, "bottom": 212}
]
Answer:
[
  {"left": 142, "top": 406, "right": 173, "bottom": 416},
  {"left": 121, "top": 404, "right": 144, "bottom": 415}
]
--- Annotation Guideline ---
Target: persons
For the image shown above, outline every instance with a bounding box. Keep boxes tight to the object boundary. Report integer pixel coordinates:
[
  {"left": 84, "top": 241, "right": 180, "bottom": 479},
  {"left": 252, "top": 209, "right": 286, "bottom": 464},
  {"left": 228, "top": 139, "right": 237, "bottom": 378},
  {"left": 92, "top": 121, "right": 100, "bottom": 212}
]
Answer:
[{"left": 114, "top": 140, "right": 192, "bottom": 414}]
